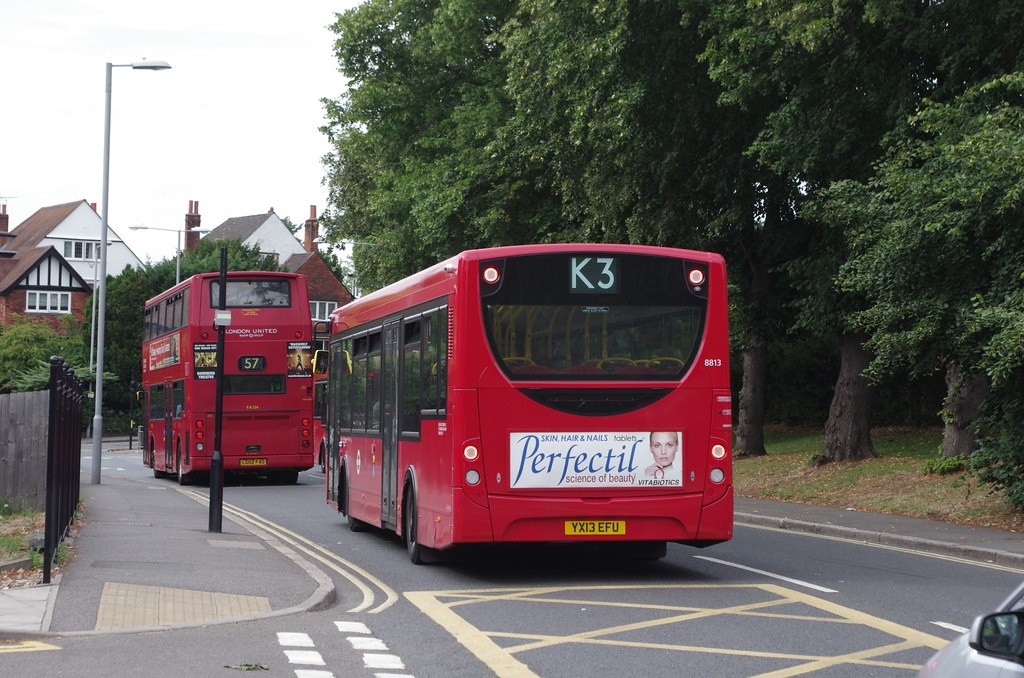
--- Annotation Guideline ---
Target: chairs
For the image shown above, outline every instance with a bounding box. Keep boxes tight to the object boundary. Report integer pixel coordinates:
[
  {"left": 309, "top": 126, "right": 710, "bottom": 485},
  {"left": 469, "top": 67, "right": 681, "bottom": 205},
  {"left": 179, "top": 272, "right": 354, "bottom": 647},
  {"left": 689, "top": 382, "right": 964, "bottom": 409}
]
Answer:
[{"left": 493, "top": 347, "right": 685, "bottom": 383}]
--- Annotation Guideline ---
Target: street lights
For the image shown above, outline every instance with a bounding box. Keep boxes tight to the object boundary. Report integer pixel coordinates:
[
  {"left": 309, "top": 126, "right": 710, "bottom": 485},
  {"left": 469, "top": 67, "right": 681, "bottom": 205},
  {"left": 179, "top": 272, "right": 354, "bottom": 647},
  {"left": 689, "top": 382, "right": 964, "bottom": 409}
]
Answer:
[{"left": 88, "top": 58, "right": 174, "bottom": 484}]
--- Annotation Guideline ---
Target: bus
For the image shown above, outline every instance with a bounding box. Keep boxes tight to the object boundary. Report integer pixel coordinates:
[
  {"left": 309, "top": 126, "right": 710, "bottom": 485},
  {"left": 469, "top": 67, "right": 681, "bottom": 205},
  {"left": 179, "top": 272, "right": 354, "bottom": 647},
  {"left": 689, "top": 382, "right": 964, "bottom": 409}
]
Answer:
[
  {"left": 140, "top": 269, "right": 316, "bottom": 487},
  {"left": 311, "top": 371, "right": 328, "bottom": 474},
  {"left": 311, "top": 244, "right": 735, "bottom": 573}
]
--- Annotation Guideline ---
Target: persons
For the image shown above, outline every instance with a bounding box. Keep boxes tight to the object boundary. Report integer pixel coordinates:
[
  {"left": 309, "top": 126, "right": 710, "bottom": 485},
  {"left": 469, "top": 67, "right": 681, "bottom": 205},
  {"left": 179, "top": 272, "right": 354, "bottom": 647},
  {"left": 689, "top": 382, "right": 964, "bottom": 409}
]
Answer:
[
  {"left": 295, "top": 352, "right": 303, "bottom": 371},
  {"left": 645, "top": 432, "right": 679, "bottom": 479}
]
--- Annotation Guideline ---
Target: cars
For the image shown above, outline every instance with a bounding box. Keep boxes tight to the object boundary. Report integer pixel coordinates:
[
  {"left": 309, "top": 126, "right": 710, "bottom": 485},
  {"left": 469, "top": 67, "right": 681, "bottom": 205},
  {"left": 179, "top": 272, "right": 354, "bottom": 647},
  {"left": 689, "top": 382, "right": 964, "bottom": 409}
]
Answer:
[{"left": 919, "top": 577, "right": 1024, "bottom": 678}]
